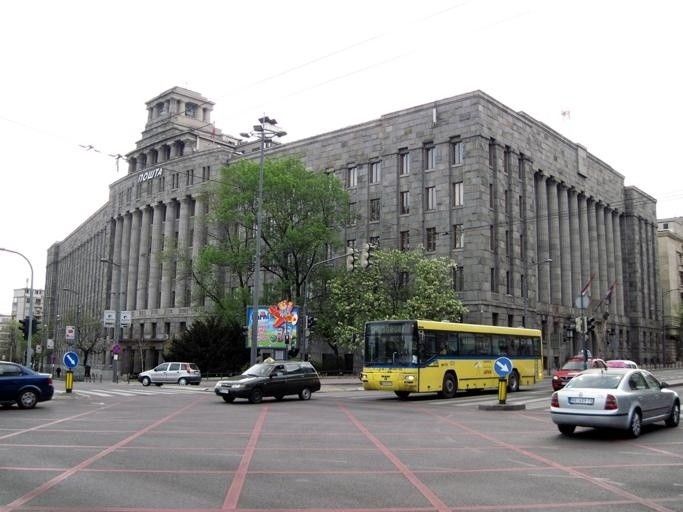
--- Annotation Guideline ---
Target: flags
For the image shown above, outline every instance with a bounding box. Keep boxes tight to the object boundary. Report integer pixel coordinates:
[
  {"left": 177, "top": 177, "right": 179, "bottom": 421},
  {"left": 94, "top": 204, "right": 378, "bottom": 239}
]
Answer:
[
  {"left": 604, "top": 287, "right": 614, "bottom": 303},
  {"left": 581, "top": 276, "right": 594, "bottom": 297}
]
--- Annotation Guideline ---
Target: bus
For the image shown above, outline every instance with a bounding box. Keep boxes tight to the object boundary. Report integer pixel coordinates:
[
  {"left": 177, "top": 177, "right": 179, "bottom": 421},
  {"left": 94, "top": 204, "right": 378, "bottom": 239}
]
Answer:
[{"left": 361, "top": 320, "right": 544, "bottom": 398}]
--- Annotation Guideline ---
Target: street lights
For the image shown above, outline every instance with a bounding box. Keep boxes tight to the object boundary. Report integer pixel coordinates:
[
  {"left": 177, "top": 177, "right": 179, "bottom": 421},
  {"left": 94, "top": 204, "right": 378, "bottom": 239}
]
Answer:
[
  {"left": 240, "top": 112, "right": 287, "bottom": 367},
  {"left": 524, "top": 258, "right": 553, "bottom": 328},
  {"left": 63, "top": 288, "right": 80, "bottom": 345},
  {"left": 662, "top": 285, "right": 683, "bottom": 367},
  {"left": 0, "top": 248, "right": 33, "bottom": 370},
  {"left": 101, "top": 258, "right": 121, "bottom": 382}
]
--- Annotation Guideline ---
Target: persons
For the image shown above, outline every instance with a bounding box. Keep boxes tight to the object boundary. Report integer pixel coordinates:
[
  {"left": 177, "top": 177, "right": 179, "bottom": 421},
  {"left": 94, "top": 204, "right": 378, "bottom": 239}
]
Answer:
[{"left": 439, "top": 342, "right": 447, "bottom": 355}]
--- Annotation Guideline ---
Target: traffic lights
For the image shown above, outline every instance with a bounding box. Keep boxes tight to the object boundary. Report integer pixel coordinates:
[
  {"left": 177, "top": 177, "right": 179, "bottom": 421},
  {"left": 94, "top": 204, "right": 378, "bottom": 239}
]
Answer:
[
  {"left": 285, "top": 334, "right": 289, "bottom": 344},
  {"left": 566, "top": 317, "right": 595, "bottom": 331},
  {"left": 347, "top": 243, "right": 375, "bottom": 272},
  {"left": 18, "top": 316, "right": 42, "bottom": 340}
]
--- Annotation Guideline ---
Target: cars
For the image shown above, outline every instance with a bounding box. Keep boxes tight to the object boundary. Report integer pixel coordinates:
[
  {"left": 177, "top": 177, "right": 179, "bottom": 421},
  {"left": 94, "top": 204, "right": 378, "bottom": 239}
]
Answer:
[
  {"left": 0, "top": 361, "right": 54, "bottom": 409},
  {"left": 550, "top": 357, "right": 680, "bottom": 436},
  {"left": 214, "top": 362, "right": 320, "bottom": 403},
  {"left": 138, "top": 361, "right": 201, "bottom": 386}
]
